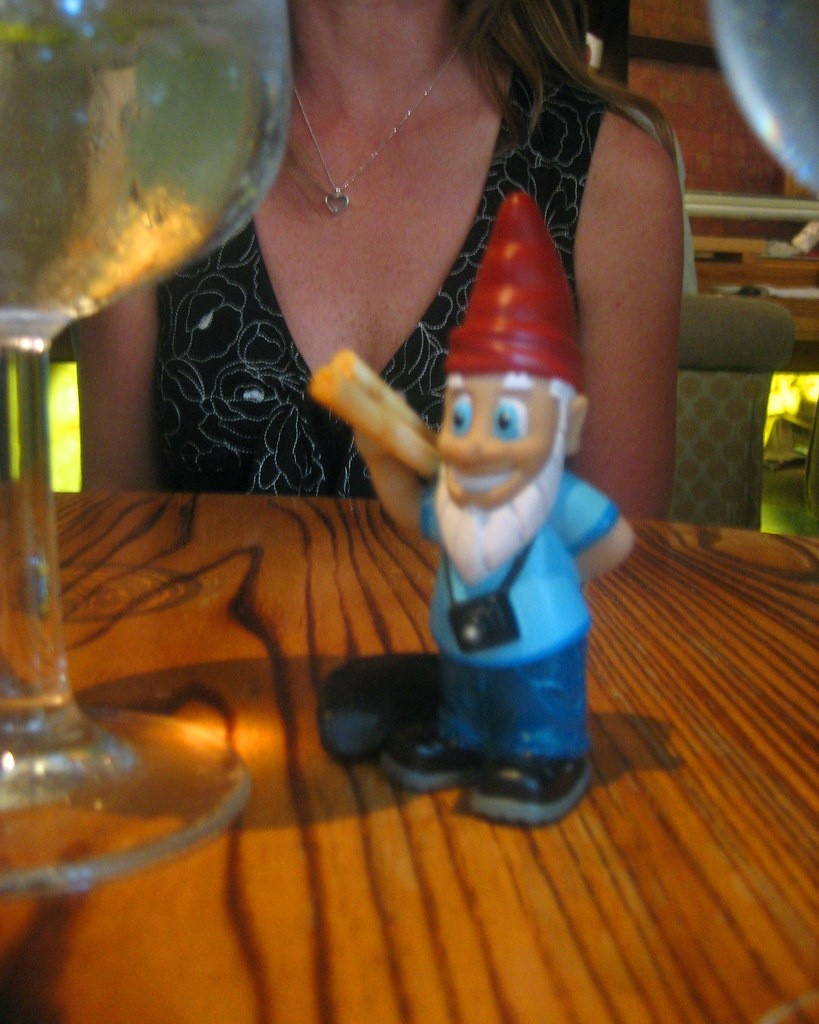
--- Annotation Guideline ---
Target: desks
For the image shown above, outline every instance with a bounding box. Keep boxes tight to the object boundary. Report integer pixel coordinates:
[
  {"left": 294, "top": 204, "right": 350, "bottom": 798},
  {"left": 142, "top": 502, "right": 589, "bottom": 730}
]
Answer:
[{"left": 0, "top": 491, "right": 819, "bottom": 1024}]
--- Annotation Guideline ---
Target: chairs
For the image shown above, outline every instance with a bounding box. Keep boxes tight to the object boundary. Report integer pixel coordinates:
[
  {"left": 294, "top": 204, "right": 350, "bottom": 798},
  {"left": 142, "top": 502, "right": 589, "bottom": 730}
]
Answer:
[{"left": 669, "top": 292, "right": 794, "bottom": 529}]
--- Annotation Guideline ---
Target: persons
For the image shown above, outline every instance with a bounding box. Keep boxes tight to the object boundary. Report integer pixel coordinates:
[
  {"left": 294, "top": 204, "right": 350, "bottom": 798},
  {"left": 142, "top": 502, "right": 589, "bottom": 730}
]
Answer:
[
  {"left": 355, "top": 192, "right": 643, "bottom": 828},
  {"left": 77, "top": 2, "right": 686, "bottom": 514}
]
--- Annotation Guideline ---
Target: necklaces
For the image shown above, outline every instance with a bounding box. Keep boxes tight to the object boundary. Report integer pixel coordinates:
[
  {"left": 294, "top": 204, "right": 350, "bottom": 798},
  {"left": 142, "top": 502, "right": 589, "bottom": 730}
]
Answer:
[{"left": 292, "top": 40, "right": 463, "bottom": 214}]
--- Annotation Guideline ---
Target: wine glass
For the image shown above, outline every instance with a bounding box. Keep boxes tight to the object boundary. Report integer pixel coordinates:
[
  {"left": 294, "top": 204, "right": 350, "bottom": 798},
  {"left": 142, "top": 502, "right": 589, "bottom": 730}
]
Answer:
[{"left": 0, "top": 0, "right": 293, "bottom": 900}]
